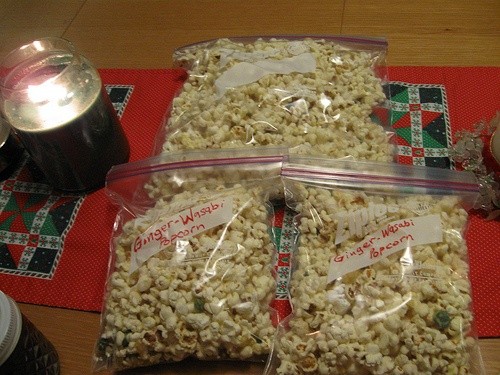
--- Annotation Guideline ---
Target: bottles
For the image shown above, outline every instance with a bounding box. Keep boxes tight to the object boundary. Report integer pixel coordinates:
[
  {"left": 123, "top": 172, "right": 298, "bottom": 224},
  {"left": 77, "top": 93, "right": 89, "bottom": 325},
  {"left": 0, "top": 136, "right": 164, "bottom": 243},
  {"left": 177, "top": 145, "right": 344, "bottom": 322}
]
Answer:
[{"left": 1, "top": 291, "right": 59, "bottom": 375}]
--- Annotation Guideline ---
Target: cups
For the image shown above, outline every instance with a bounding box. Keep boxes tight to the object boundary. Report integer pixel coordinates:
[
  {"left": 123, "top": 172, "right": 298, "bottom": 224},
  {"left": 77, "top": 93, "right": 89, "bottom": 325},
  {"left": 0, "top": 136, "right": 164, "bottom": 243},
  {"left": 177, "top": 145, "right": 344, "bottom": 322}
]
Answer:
[{"left": 0, "top": 37, "right": 131, "bottom": 195}]
